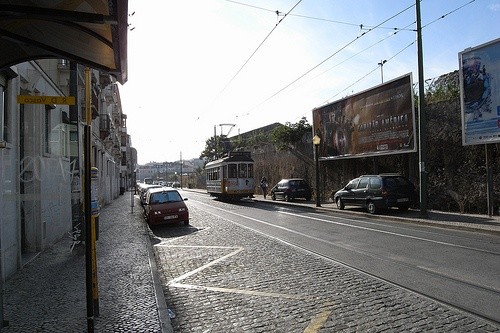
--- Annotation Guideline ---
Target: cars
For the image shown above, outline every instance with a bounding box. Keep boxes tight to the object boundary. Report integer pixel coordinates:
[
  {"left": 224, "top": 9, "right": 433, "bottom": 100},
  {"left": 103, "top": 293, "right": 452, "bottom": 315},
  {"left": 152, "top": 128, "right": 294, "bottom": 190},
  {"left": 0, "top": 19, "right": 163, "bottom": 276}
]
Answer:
[
  {"left": 137, "top": 182, "right": 163, "bottom": 205},
  {"left": 143, "top": 187, "right": 190, "bottom": 229},
  {"left": 158, "top": 181, "right": 181, "bottom": 188}
]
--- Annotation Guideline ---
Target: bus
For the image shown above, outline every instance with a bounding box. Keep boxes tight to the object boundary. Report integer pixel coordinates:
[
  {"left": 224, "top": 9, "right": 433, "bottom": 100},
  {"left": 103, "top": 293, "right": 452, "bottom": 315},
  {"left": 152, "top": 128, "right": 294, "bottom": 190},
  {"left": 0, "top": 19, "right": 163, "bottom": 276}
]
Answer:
[
  {"left": 144, "top": 178, "right": 153, "bottom": 185},
  {"left": 205, "top": 151, "right": 256, "bottom": 201}
]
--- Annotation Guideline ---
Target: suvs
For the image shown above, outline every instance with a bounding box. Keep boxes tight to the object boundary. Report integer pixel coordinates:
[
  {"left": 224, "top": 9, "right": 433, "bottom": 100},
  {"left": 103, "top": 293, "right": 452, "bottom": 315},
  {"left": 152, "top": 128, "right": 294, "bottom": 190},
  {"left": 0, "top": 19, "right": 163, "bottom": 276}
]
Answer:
[
  {"left": 333, "top": 174, "right": 417, "bottom": 214},
  {"left": 270, "top": 178, "right": 312, "bottom": 202}
]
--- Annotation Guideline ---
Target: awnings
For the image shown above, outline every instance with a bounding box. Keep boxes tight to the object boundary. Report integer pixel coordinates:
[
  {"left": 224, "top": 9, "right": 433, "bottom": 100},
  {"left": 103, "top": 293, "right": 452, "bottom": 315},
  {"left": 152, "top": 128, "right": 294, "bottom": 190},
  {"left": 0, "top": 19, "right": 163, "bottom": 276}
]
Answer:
[{"left": 0, "top": 0, "right": 128, "bottom": 84}]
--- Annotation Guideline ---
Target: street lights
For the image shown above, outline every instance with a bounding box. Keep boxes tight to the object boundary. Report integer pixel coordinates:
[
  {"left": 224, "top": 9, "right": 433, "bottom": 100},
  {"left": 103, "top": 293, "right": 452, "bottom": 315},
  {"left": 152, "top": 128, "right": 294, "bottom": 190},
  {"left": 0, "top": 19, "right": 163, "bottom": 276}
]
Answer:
[{"left": 313, "top": 132, "right": 321, "bottom": 207}]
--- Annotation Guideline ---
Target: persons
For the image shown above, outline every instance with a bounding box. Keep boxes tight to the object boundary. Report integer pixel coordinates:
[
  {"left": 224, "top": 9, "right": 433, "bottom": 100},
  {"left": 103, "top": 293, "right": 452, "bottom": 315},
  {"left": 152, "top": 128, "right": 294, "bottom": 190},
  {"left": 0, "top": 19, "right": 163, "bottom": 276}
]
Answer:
[
  {"left": 260, "top": 176, "right": 268, "bottom": 199},
  {"left": 315, "top": 111, "right": 357, "bottom": 157}
]
